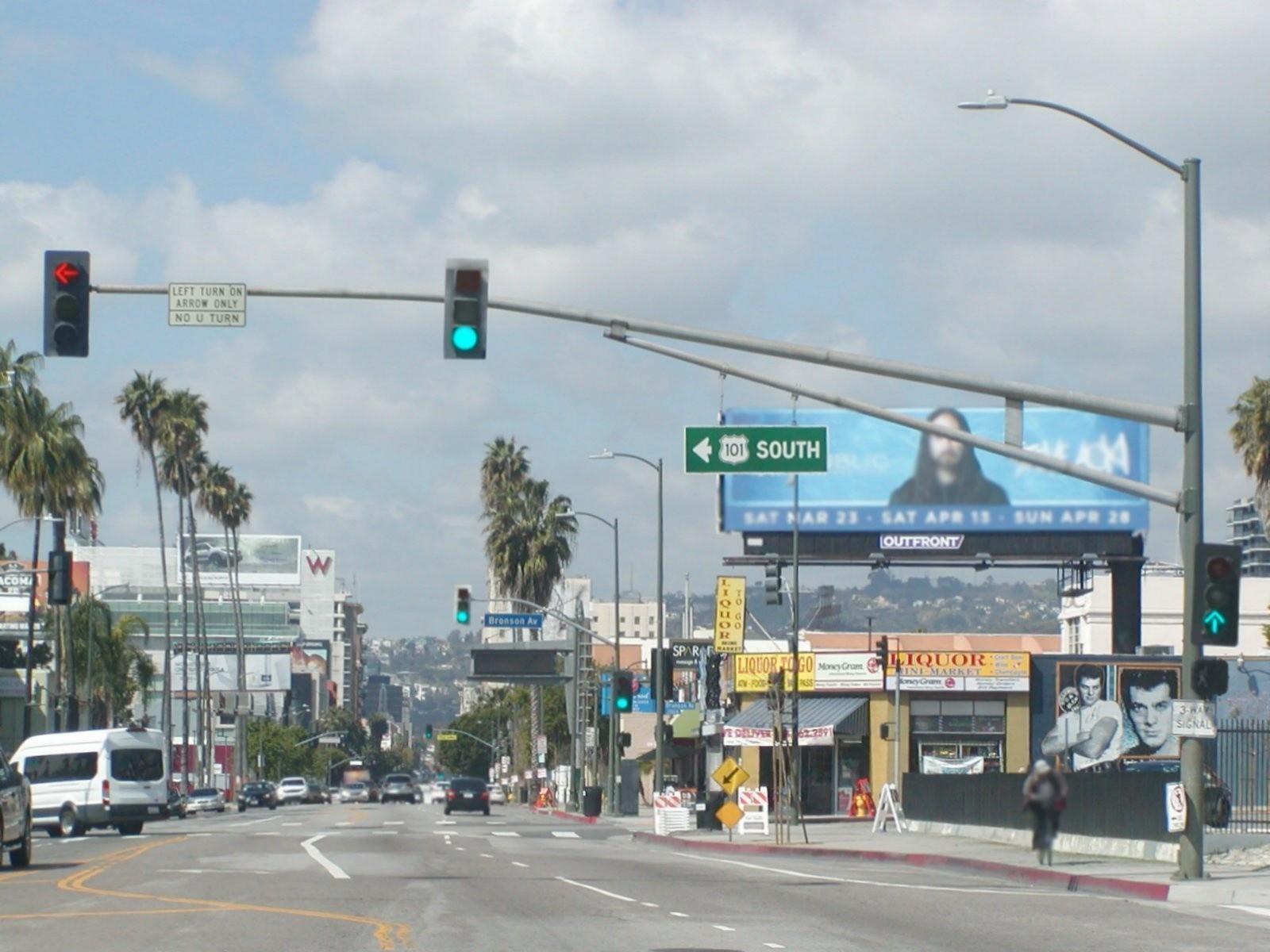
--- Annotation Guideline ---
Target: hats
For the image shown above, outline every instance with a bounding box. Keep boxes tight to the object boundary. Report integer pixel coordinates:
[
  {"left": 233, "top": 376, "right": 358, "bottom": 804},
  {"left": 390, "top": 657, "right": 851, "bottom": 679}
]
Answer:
[{"left": 1034, "top": 760, "right": 1052, "bottom": 775}]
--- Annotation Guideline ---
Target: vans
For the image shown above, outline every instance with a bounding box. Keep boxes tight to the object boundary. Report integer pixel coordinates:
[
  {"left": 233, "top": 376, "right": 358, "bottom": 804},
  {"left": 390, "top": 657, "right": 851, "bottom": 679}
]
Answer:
[{"left": 7, "top": 720, "right": 169, "bottom": 840}]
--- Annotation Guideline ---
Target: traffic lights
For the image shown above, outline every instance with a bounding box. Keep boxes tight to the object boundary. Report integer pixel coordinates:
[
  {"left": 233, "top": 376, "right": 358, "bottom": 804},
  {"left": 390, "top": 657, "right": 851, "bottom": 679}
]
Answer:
[
  {"left": 764, "top": 560, "right": 783, "bottom": 606},
  {"left": 441, "top": 258, "right": 490, "bottom": 361},
  {"left": 425, "top": 725, "right": 433, "bottom": 739},
  {"left": 1191, "top": 541, "right": 1242, "bottom": 647},
  {"left": 875, "top": 634, "right": 889, "bottom": 670},
  {"left": 453, "top": 584, "right": 473, "bottom": 626},
  {"left": 42, "top": 250, "right": 89, "bottom": 357},
  {"left": 682, "top": 423, "right": 831, "bottom": 473},
  {"left": 1191, "top": 656, "right": 1230, "bottom": 697},
  {"left": 612, "top": 670, "right": 633, "bottom": 713}
]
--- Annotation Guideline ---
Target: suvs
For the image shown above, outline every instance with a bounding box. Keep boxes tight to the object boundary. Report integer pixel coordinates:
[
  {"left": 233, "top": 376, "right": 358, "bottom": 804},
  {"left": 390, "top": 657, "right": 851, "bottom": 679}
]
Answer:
[{"left": 183, "top": 540, "right": 244, "bottom": 568}]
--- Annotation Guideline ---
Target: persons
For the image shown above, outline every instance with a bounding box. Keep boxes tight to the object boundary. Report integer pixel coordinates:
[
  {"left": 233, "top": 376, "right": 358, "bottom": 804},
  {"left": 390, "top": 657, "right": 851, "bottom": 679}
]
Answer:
[
  {"left": 1038, "top": 661, "right": 1123, "bottom": 773},
  {"left": 1021, "top": 760, "right": 1069, "bottom": 866},
  {"left": 888, "top": 404, "right": 1011, "bottom": 508},
  {"left": 1118, "top": 669, "right": 1183, "bottom": 761}
]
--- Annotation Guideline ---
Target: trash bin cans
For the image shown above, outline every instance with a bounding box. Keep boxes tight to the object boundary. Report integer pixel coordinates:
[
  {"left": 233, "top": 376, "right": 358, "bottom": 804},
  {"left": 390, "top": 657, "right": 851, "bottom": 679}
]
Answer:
[
  {"left": 694, "top": 791, "right": 725, "bottom": 831},
  {"left": 582, "top": 786, "right": 603, "bottom": 816},
  {"left": 520, "top": 786, "right": 528, "bottom": 803}
]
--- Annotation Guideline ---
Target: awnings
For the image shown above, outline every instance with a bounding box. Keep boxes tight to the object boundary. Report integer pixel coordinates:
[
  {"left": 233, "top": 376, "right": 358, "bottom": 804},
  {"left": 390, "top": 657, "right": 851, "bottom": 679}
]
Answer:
[
  {"left": 666, "top": 704, "right": 713, "bottom": 739},
  {"left": 718, "top": 698, "right": 870, "bottom": 744}
]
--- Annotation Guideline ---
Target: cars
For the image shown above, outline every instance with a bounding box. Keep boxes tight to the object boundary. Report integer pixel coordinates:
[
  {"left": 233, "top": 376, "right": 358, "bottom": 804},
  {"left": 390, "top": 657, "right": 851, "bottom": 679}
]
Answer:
[
  {"left": 182, "top": 786, "right": 227, "bottom": 815},
  {"left": 163, "top": 786, "right": 187, "bottom": 820},
  {"left": 442, "top": 776, "right": 493, "bottom": 816},
  {"left": 233, "top": 771, "right": 416, "bottom": 812},
  {"left": 486, "top": 783, "right": 509, "bottom": 806},
  {"left": 1122, "top": 759, "right": 1233, "bottom": 829},
  {"left": 413, "top": 778, "right": 450, "bottom": 804},
  {"left": 0, "top": 746, "right": 33, "bottom": 868}
]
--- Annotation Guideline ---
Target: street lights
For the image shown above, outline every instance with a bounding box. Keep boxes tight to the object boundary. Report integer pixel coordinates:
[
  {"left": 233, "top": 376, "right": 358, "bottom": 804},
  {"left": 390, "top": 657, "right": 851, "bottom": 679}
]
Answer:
[
  {"left": 555, "top": 507, "right": 620, "bottom": 817},
  {"left": 954, "top": 94, "right": 1209, "bottom": 884},
  {"left": 587, "top": 446, "right": 665, "bottom": 796},
  {"left": 82, "top": 582, "right": 132, "bottom": 734},
  {"left": 886, "top": 637, "right": 901, "bottom": 796}
]
